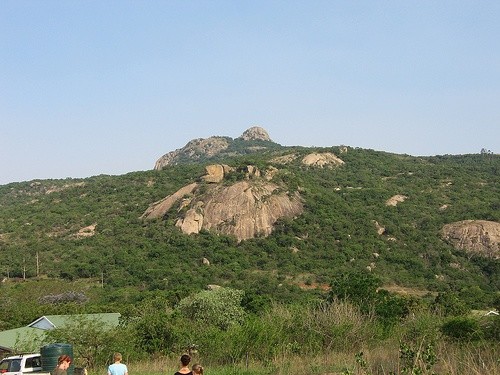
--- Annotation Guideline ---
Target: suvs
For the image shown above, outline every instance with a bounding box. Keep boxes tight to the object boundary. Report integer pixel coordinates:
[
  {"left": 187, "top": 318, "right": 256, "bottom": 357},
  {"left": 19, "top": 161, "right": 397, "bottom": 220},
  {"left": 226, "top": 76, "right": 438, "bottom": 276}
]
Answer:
[{"left": 0, "top": 353, "right": 42, "bottom": 375}]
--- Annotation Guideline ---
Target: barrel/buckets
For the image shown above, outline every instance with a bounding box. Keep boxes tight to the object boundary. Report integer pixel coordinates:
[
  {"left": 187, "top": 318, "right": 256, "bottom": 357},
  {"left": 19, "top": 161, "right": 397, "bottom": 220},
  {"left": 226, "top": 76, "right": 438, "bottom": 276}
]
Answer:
[{"left": 40, "top": 344, "right": 74, "bottom": 375}]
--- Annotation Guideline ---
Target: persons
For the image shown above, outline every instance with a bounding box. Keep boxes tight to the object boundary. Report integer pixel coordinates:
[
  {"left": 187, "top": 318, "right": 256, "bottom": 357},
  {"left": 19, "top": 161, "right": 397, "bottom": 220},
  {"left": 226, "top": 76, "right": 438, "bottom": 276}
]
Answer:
[
  {"left": 51, "top": 354, "right": 71, "bottom": 375},
  {"left": 175, "top": 354, "right": 193, "bottom": 375},
  {"left": 192, "top": 364, "right": 204, "bottom": 375},
  {"left": 108, "top": 353, "right": 129, "bottom": 375}
]
